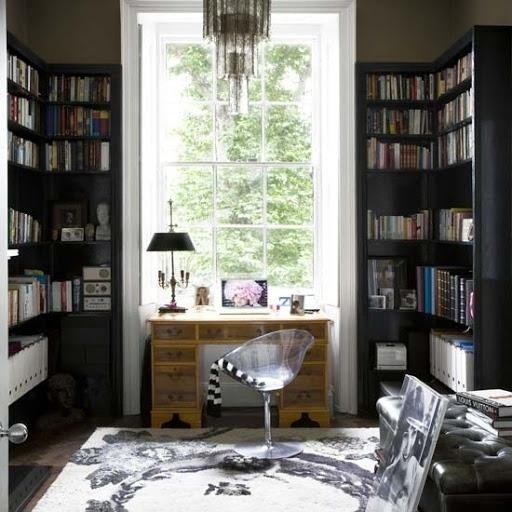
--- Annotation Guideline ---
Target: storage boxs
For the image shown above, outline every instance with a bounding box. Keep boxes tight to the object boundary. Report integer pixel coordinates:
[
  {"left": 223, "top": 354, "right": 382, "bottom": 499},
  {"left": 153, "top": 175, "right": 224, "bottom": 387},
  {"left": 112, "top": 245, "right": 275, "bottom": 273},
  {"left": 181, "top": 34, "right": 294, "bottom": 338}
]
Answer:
[{"left": 8, "top": 335, "right": 50, "bottom": 407}]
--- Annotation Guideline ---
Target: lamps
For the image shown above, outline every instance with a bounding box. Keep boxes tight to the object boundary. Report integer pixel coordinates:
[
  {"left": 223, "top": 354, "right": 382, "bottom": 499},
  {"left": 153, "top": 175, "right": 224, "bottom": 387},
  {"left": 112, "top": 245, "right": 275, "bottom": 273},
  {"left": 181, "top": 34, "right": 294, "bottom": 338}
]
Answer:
[
  {"left": 202, "top": 0, "right": 272, "bottom": 116},
  {"left": 146, "top": 199, "right": 198, "bottom": 313}
]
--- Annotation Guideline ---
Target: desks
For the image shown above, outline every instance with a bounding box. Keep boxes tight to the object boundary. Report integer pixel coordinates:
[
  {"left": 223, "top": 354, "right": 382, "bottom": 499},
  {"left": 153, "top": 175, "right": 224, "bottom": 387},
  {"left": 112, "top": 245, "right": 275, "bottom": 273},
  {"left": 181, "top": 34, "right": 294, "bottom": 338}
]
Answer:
[{"left": 147, "top": 309, "right": 334, "bottom": 428}]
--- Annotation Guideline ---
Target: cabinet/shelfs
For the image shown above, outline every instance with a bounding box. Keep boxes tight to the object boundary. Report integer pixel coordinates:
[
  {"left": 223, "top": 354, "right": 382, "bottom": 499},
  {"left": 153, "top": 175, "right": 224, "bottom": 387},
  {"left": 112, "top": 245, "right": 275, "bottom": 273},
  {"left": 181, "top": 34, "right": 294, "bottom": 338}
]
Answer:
[
  {"left": 422, "top": 24, "right": 512, "bottom": 401},
  {"left": 7, "top": 29, "right": 52, "bottom": 456},
  {"left": 53, "top": 64, "right": 123, "bottom": 421},
  {"left": 356, "top": 60, "right": 430, "bottom": 424}
]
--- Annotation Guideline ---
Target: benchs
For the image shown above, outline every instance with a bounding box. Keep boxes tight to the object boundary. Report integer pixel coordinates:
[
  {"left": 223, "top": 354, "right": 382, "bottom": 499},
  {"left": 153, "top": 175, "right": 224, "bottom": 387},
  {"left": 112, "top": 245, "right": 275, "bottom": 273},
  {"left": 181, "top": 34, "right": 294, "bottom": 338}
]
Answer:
[{"left": 377, "top": 387, "right": 511, "bottom": 512}]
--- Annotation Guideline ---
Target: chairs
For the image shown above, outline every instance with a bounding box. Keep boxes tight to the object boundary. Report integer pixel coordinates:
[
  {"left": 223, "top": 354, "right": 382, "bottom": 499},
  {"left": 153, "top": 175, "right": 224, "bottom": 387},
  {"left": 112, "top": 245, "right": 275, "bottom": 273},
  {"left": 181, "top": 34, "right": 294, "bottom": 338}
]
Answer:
[{"left": 218, "top": 327, "right": 316, "bottom": 459}]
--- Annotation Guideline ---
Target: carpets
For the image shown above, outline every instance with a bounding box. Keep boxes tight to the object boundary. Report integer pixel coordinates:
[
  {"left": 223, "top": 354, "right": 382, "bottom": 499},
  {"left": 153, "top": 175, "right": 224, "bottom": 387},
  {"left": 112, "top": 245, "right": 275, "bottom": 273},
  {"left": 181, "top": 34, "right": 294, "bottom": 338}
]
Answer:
[{"left": 31, "top": 425, "right": 383, "bottom": 512}]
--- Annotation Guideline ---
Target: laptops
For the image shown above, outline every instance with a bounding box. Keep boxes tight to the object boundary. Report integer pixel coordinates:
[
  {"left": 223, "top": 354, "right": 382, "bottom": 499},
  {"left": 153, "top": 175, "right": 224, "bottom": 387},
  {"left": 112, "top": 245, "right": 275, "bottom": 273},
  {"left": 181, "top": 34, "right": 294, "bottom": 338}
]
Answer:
[{"left": 220, "top": 278, "right": 269, "bottom": 315}]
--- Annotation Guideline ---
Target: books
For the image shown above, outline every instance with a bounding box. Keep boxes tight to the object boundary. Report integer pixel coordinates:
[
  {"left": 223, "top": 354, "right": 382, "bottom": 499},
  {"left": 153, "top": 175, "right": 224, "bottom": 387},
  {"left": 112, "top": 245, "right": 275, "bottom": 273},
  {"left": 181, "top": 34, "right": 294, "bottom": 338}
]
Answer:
[
  {"left": 428, "top": 328, "right": 512, "bottom": 437},
  {"left": 365, "top": 50, "right": 474, "bottom": 326},
  {"left": 362, "top": 374, "right": 450, "bottom": 512},
  {"left": 7, "top": 48, "right": 112, "bottom": 408}
]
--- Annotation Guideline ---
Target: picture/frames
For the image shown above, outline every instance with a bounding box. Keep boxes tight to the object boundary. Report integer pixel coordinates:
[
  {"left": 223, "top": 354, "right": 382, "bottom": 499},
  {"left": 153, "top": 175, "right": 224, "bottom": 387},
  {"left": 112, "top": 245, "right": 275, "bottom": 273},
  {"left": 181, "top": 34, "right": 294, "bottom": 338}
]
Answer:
[{"left": 53, "top": 196, "right": 89, "bottom": 231}]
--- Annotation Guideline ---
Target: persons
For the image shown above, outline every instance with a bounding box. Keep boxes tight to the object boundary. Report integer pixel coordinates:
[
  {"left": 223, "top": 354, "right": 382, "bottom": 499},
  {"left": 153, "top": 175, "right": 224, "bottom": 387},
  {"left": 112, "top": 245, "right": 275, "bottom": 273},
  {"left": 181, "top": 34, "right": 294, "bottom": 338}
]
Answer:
[
  {"left": 291, "top": 300, "right": 302, "bottom": 314},
  {"left": 224, "top": 279, "right": 264, "bottom": 307},
  {"left": 196, "top": 287, "right": 209, "bottom": 306}
]
